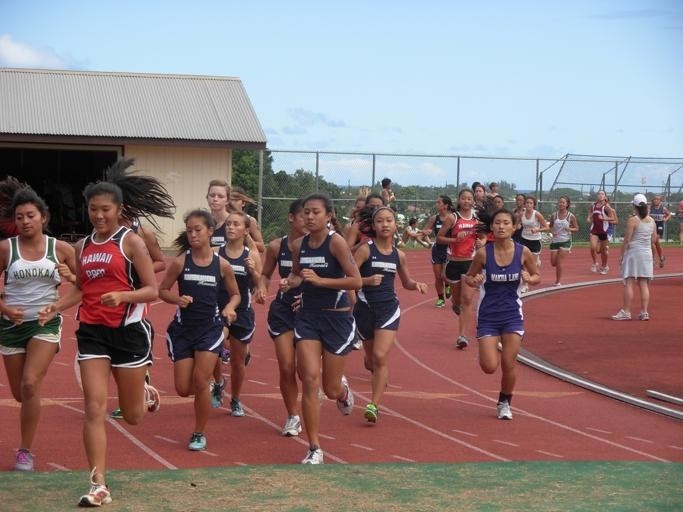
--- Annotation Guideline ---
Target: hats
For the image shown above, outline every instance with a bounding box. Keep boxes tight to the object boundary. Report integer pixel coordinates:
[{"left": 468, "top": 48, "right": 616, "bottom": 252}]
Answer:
[{"left": 631, "top": 193, "right": 648, "bottom": 207}]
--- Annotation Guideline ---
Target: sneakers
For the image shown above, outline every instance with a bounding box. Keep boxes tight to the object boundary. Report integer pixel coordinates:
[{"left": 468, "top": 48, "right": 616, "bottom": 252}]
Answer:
[
  {"left": 590, "top": 260, "right": 609, "bottom": 274},
  {"left": 336, "top": 374, "right": 354, "bottom": 415},
  {"left": 216, "top": 347, "right": 253, "bottom": 366},
  {"left": 301, "top": 445, "right": 325, "bottom": 465},
  {"left": 188, "top": 432, "right": 206, "bottom": 451},
  {"left": 207, "top": 374, "right": 227, "bottom": 408},
  {"left": 364, "top": 402, "right": 379, "bottom": 423},
  {"left": 496, "top": 401, "right": 514, "bottom": 421},
  {"left": 111, "top": 383, "right": 162, "bottom": 419},
  {"left": 436, "top": 280, "right": 470, "bottom": 350},
  {"left": 14, "top": 446, "right": 35, "bottom": 472},
  {"left": 659, "top": 256, "right": 666, "bottom": 268},
  {"left": 611, "top": 306, "right": 650, "bottom": 321},
  {"left": 230, "top": 395, "right": 245, "bottom": 417},
  {"left": 280, "top": 413, "right": 302, "bottom": 436},
  {"left": 77, "top": 484, "right": 112, "bottom": 507},
  {"left": 518, "top": 279, "right": 563, "bottom": 294}
]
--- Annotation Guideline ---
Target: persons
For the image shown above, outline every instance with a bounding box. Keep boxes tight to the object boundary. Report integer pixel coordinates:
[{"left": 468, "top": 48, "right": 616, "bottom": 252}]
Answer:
[{"left": 1, "top": 159, "right": 683, "bottom": 507}]
